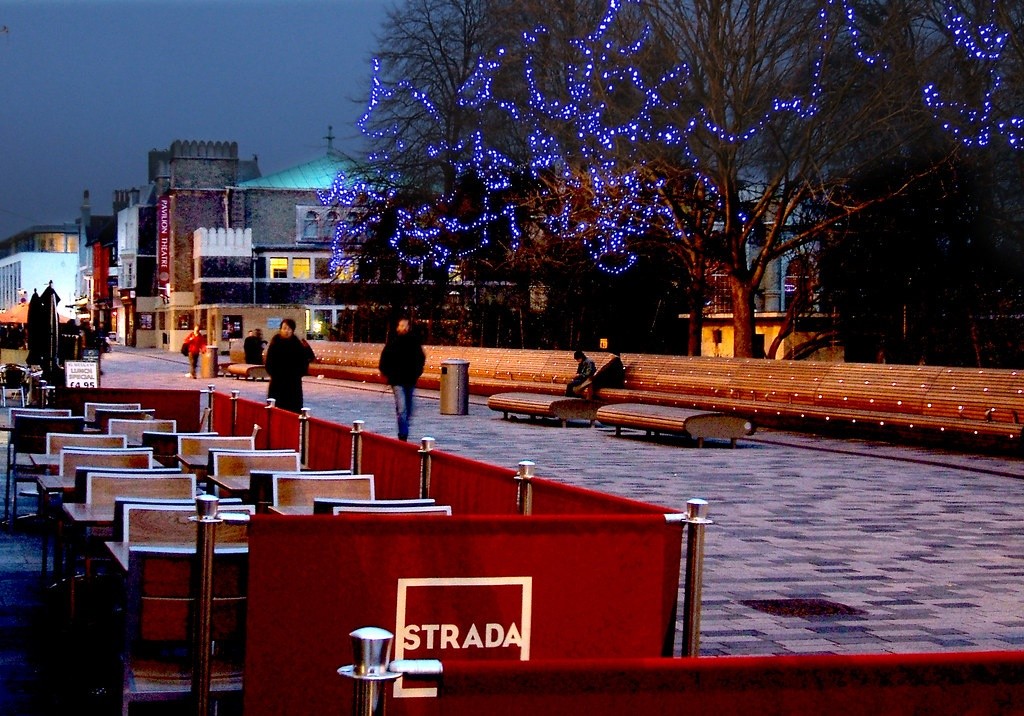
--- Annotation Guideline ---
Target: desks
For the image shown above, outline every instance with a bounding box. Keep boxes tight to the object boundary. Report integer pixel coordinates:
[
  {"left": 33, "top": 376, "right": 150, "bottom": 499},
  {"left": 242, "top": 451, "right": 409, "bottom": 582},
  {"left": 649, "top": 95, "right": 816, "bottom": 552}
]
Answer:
[
  {"left": 101, "top": 540, "right": 250, "bottom": 656},
  {"left": 62, "top": 501, "right": 116, "bottom": 644},
  {"left": 268, "top": 502, "right": 315, "bottom": 516},
  {"left": 125, "top": 436, "right": 142, "bottom": 447},
  {"left": 83, "top": 417, "right": 156, "bottom": 423},
  {"left": 205, "top": 474, "right": 250, "bottom": 498},
  {"left": 28, "top": 453, "right": 165, "bottom": 578},
  {"left": 0, "top": 425, "right": 102, "bottom": 527},
  {"left": 175, "top": 454, "right": 208, "bottom": 469}
]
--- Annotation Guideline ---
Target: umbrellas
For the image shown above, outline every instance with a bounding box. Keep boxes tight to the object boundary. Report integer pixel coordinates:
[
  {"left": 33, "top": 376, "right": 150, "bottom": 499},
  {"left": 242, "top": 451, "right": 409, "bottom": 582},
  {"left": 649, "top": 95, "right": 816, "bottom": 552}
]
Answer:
[{"left": 0, "top": 280, "right": 70, "bottom": 384}]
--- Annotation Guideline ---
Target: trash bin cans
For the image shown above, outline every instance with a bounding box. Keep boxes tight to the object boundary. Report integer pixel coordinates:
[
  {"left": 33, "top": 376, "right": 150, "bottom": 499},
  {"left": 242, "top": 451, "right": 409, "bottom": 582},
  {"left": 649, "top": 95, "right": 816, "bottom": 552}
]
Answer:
[
  {"left": 439, "top": 358, "right": 471, "bottom": 416},
  {"left": 200, "top": 345, "right": 220, "bottom": 379}
]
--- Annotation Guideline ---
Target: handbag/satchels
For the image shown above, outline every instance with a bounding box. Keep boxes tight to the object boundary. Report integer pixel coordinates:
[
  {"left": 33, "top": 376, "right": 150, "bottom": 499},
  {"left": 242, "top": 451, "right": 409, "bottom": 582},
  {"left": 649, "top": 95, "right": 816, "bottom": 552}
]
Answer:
[{"left": 180, "top": 342, "right": 190, "bottom": 356}]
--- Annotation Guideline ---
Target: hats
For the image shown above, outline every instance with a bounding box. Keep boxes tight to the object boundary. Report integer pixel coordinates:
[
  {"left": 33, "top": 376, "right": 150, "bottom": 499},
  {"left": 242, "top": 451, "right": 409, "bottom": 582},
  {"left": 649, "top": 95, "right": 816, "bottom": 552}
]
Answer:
[{"left": 608, "top": 349, "right": 621, "bottom": 356}]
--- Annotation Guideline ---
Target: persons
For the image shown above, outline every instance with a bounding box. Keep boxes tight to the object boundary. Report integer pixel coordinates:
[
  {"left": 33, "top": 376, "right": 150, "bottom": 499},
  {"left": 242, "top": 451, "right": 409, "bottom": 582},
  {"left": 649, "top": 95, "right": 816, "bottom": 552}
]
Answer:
[
  {"left": 566, "top": 350, "right": 595, "bottom": 397},
  {"left": 243, "top": 330, "right": 262, "bottom": 365},
  {"left": 184, "top": 324, "right": 206, "bottom": 379},
  {"left": 301, "top": 338, "right": 315, "bottom": 362},
  {"left": 378, "top": 315, "right": 426, "bottom": 441},
  {"left": 573, "top": 349, "right": 624, "bottom": 401},
  {"left": 265, "top": 319, "right": 309, "bottom": 414}
]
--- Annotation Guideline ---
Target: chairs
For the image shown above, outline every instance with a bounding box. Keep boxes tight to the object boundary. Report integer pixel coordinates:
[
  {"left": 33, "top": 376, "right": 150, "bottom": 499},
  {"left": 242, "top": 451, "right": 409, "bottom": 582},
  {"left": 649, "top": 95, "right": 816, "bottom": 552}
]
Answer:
[{"left": 7, "top": 403, "right": 452, "bottom": 716}]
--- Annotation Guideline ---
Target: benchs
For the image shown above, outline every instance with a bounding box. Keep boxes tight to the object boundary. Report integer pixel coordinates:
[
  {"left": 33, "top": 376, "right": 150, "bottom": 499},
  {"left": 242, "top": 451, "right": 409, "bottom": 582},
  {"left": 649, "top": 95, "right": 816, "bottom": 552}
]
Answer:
[
  {"left": 218, "top": 362, "right": 239, "bottom": 378},
  {"left": 228, "top": 364, "right": 269, "bottom": 382},
  {"left": 488, "top": 393, "right": 599, "bottom": 427},
  {"left": 230, "top": 337, "right": 1024, "bottom": 459},
  {"left": 598, "top": 403, "right": 756, "bottom": 448}
]
